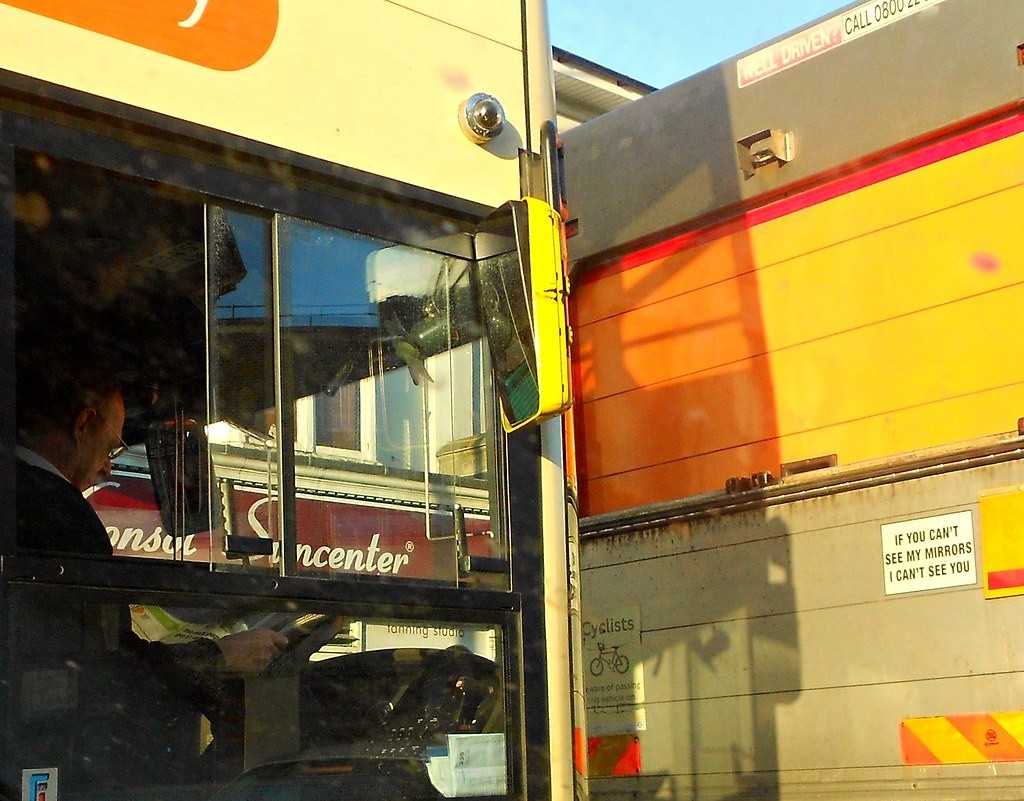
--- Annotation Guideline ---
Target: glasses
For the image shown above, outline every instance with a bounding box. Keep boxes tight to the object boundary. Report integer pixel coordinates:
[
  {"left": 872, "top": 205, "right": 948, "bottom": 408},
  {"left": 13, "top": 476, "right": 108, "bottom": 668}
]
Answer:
[{"left": 95, "top": 412, "right": 129, "bottom": 459}]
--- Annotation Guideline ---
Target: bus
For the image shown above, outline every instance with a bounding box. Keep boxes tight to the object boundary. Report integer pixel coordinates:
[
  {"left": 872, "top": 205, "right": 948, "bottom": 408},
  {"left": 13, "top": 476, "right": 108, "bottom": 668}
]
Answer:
[{"left": 2, "top": 0, "right": 590, "bottom": 801}]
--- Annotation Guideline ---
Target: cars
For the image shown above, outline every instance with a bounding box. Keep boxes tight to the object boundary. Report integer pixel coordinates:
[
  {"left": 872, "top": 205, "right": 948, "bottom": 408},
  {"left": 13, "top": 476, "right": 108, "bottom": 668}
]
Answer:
[{"left": 205, "top": 743, "right": 442, "bottom": 801}]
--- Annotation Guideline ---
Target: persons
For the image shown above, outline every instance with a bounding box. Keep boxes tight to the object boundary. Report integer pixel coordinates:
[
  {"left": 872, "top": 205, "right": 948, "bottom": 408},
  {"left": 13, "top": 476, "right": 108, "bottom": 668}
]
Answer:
[{"left": 15, "top": 344, "right": 289, "bottom": 778}]
